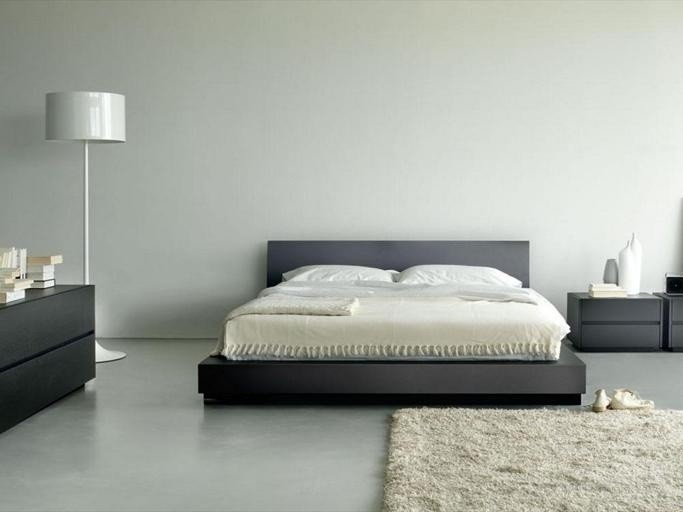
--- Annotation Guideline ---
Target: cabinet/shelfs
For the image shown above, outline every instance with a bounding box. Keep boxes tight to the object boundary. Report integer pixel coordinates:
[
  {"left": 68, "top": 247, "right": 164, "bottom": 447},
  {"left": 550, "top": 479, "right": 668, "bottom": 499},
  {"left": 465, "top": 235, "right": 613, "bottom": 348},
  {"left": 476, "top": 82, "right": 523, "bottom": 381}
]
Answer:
[{"left": 1, "top": 285, "right": 97, "bottom": 433}]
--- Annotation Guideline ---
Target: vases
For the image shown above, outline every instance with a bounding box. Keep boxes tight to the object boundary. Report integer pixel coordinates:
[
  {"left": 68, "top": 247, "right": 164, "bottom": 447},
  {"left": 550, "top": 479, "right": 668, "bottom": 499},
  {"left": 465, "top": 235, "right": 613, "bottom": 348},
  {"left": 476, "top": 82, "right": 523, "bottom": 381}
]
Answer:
[{"left": 603, "top": 234, "right": 643, "bottom": 294}]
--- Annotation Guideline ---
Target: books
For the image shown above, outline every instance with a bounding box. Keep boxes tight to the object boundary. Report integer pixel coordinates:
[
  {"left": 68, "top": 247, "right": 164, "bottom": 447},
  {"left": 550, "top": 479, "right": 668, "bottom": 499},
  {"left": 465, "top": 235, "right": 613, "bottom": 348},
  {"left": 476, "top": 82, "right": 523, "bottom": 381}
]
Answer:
[
  {"left": 587, "top": 282, "right": 627, "bottom": 298},
  {"left": 0, "top": 245, "right": 64, "bottom": 304}
]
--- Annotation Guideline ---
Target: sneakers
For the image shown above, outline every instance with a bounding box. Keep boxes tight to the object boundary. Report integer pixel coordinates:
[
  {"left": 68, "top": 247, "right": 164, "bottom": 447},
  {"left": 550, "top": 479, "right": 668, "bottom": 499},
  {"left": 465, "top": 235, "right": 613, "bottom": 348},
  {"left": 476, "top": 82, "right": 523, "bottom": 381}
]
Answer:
[
  {"left": 592, "top": 388, "right": 612, "bottom": 412},
  {"left": 610, "top": 388, "right": 655, "bottom": 410}
]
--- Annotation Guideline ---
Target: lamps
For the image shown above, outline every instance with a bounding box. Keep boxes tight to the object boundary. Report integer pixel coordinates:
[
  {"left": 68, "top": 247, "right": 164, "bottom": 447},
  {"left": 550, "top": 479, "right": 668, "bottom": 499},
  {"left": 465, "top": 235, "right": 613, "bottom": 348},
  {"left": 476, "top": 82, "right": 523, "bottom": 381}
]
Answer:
[{"left": 46, "top": 92, "right": 129, "bottom": 363}]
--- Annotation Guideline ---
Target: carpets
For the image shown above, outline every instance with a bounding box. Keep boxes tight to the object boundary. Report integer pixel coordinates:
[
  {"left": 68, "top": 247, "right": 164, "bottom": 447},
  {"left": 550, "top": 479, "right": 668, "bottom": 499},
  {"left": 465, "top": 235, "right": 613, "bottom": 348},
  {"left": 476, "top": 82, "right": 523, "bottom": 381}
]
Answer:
[{"left": 381, "top": 409, "right": 682, "bottom": 512}]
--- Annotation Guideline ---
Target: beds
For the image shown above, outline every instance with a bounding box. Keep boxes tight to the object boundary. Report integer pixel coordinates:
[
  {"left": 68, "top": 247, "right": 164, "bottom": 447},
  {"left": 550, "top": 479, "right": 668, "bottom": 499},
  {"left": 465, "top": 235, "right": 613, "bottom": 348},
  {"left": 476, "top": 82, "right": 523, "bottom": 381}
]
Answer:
[{"left": 199, "top": 240, "right": 587, "bottom": 407}]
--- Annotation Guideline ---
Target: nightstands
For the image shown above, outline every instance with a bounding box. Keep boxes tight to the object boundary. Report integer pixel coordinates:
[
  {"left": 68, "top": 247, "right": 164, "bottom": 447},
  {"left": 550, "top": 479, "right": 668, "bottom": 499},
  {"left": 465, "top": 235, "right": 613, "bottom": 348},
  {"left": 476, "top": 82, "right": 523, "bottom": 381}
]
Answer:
[{"left": 567, "top": 292, "right": 682, "bottom": 353}]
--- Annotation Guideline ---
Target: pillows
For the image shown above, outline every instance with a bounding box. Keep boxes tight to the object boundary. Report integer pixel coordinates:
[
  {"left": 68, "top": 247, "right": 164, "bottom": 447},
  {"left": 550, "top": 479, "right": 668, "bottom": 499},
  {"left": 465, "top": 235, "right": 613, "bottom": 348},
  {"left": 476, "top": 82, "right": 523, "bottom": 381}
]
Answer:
[{"left": 282, "top": 265, "right": 522, "bottom": 285}]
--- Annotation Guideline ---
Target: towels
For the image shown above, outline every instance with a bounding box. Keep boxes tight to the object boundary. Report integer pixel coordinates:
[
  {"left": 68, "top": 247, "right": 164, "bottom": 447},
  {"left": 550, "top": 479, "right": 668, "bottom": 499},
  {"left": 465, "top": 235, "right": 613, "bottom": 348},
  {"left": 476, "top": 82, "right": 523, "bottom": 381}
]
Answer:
[{"left": 210, "top": 294, "right": 358, "bottom": 359}]
